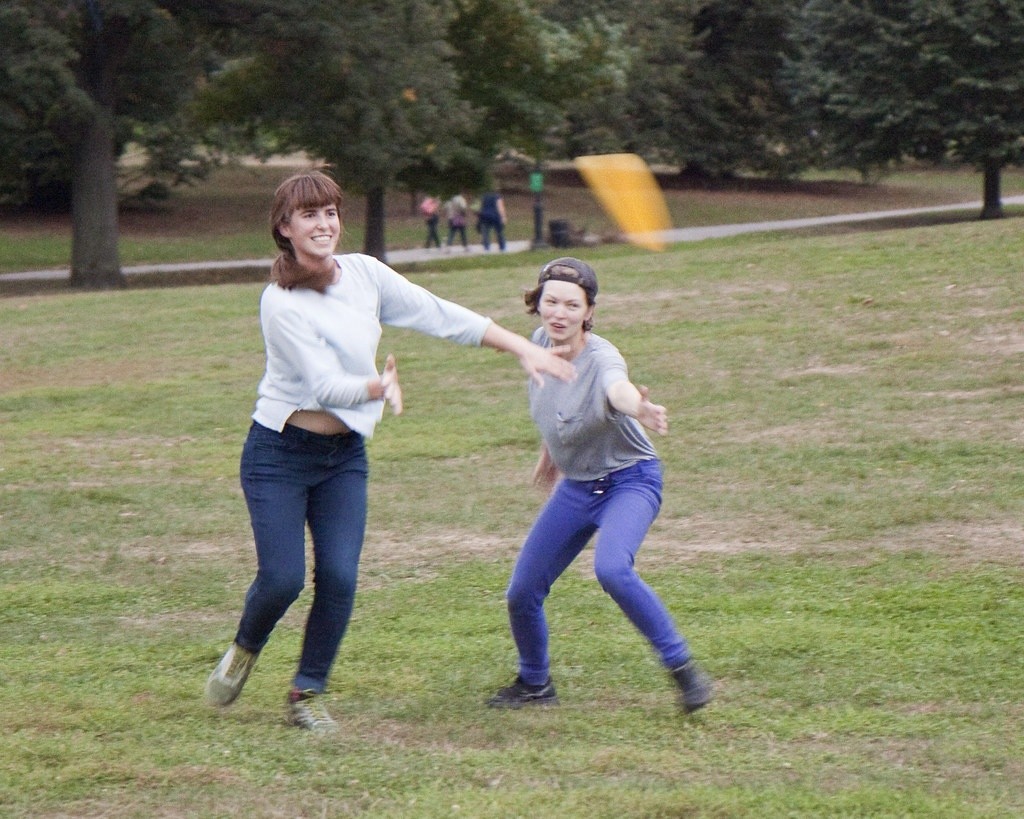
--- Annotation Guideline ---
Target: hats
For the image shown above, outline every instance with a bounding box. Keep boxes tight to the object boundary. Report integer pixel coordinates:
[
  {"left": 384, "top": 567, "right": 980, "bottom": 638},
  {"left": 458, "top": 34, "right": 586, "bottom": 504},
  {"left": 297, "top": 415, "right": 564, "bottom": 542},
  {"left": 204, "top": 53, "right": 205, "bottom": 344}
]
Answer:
[{"left": 539, "top": 256, "right": 598, "bottom": 294}]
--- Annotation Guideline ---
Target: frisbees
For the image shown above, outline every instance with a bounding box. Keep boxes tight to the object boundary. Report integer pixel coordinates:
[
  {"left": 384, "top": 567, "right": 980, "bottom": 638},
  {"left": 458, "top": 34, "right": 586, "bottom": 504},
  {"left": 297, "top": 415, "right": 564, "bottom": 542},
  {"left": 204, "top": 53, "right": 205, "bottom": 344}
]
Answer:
[{"left": 571, "top": 152, "right": 674, "bottom": 252}]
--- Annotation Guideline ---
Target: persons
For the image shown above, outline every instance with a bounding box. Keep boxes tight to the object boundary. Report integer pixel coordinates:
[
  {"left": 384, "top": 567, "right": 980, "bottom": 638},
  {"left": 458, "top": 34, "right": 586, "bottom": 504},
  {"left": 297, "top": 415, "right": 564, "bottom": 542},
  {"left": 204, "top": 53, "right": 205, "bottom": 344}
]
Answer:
[
  {"left": 445, "top": 195, "right": 470, "bottom": 253},
  {"left": 487, "top": 252, "right": 716, "bottom": 712},
  {"left": 201, "top": 173, "right": 576, "bottom": 732},
  {"left": 421, "top": 195, "right": 441, "bottom": 249},
  {"left": 477, "top": 192, "right": 507, "bottom": 252}
]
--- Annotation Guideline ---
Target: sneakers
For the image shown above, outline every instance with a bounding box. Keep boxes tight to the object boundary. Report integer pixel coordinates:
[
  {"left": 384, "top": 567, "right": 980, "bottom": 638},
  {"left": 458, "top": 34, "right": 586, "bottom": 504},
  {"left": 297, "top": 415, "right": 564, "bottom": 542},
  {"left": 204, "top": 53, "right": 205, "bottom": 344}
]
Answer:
[
  {"left": 284, "top": 696, "right": 339, "bottom": 734},
  {"left": 482, "top": 680, "right": 561, "bottom": 709},
  {"left": 205, "top": 642, "right": 257, "bottom": 708},
  {"left": 674, "top": 663, "right": 713, "bottom": 712}
]
